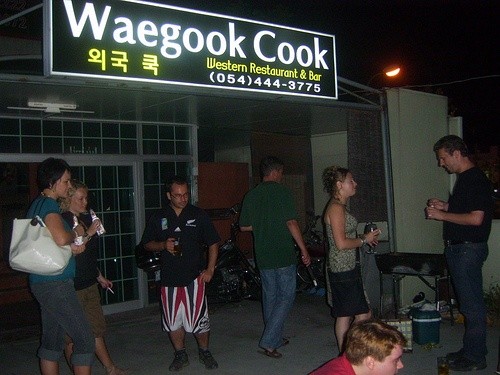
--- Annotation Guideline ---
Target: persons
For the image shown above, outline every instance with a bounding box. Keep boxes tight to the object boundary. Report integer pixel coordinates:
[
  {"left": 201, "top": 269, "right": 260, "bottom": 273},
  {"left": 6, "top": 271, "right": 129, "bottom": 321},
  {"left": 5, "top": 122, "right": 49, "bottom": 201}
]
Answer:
[
  {"left": 27, "top": 158, "right": 96, "bottom": 375},
  {"left": 322, "top": 166, "right": 380, "bottom": 354},
  {"left": 141, "top": 178, "right": 220, "bottom": 370},
  {"left": 426, "top": 135, "right": 494, "bottom": 369},
  {"left": 309, "top": 320, "right": 407, "bottom": 375},
  {"left": 62, "top": 180, "right": 130, "bottom": 375},
  {"left": 239, "top": 157, "right": 312, "bottom": 359}
]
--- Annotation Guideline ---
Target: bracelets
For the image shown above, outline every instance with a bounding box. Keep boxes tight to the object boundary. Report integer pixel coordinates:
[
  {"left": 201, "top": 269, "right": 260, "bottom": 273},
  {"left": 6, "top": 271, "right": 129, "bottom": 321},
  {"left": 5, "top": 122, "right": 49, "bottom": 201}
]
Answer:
[
  {"left": 85, "top": 233, "right": 91, "bottom": 240},
  {"left": 72, "top": 230, "right": 78, "bottom": 237},
  {"left": 359, "top": 236, "right": 367, "bottom": 246}
]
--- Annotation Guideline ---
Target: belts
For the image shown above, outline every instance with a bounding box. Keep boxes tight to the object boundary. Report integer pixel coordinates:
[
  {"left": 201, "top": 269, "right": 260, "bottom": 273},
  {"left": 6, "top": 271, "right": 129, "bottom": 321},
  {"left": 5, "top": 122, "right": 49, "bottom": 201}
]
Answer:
[{"left": 446, "top": 240, "right": 485, "bottom": 246}]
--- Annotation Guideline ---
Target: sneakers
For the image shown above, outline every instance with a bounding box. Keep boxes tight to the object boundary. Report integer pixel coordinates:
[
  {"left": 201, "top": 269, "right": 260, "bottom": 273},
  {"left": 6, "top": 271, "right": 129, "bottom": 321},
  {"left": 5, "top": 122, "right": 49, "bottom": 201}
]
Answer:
[
  {"left": 199, "top": 350, "right": 218, "bottom": 369},
  {"left": 169, "top": 350, "right": 188, "bottom": 371}
]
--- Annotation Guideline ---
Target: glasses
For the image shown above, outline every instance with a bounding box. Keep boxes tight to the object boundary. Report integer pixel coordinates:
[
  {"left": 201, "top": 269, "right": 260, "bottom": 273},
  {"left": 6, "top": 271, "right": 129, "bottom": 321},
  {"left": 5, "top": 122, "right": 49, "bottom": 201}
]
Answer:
[{"left": 170, "top": 191, "right": 189, "bottom": 198}]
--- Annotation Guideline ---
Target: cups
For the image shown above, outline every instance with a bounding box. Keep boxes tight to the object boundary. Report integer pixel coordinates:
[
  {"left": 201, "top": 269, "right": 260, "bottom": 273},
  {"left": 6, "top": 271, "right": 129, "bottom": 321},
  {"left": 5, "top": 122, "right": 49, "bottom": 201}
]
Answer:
[
  {"left": 174, "top": 240, "right": 182, "bottom": 257},
  {"left": 437, "top": 356, "right": 449, "bottom": 375}
]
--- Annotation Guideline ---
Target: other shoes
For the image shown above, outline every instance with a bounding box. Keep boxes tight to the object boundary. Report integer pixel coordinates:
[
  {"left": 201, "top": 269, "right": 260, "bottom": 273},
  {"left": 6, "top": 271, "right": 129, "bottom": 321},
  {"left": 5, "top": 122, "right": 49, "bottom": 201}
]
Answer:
[
  {"left": 495, "top": 369, "right": 500, "bottom": 375},
  {"left": 447, "top": 348, "right": 464, "bottom": 357},
  {"left": 257, "top": 345, "right": 281, "bottom": 357},
  {"left": 447, "top": 357, "right": 486, "bottom": 371},
  {"left": 107, "top": 365, "right": 130, "bottom": 375},
  {"left": 281, "top": 338, "right": 289, "bottom": 346}
]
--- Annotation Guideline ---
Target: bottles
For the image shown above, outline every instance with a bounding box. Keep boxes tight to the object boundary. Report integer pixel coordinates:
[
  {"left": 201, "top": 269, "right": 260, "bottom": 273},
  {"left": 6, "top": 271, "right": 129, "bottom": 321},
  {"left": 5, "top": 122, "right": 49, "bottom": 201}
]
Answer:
[
  {"left": 71, "top": 216, "right": 84, "bottom": 246},
  {"left": 89, "top": 209, "right": 106, "bottom": 235}
]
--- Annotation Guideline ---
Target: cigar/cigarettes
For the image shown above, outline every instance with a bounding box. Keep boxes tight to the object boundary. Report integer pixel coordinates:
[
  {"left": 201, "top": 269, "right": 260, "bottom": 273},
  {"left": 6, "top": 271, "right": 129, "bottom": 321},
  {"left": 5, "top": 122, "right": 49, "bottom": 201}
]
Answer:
[{"left": 108, "top": 287, "right": 114, "bottom": 294}]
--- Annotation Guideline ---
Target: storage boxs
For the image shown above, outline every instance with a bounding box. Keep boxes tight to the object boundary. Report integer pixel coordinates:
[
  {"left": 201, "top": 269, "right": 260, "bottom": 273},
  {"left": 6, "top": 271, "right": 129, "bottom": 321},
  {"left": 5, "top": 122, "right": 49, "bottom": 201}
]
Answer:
[
  {"left": 408, "top": 309, "right": 442, "bottom": 345},
  {"left": 381, "top": 314, "right": 412, "bottom": 353}
]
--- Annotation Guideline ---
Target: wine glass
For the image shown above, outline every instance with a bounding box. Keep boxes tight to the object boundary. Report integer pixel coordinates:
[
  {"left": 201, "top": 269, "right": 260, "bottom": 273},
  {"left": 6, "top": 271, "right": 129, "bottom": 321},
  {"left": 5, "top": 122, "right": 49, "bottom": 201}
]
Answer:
[{"left": 364, "top": 224, "right": 378, "bottom": 254}]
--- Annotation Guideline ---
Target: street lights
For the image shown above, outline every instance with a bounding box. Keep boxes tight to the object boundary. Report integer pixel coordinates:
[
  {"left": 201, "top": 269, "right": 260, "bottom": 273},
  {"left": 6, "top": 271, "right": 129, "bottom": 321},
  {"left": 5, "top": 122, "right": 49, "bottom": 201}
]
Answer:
[{"left": 365, "top": 66, "right": 401, "bottom": 104}]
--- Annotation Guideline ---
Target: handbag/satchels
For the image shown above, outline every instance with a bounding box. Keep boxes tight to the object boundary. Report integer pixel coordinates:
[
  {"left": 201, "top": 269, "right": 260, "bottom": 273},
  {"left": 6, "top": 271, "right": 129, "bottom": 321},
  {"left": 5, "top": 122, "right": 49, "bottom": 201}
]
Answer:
[
  {"left": 364, "top": 220, "right": 377, "bottom": 233},
  {"left": 135, "top": 213, "right": 167, "bottom": 272},
  {"left": 381, "top": 318, "right": 413, "bottom": 353},
  {"left": 9, "top": 197, "right": 72, "bottom": 276}
]
